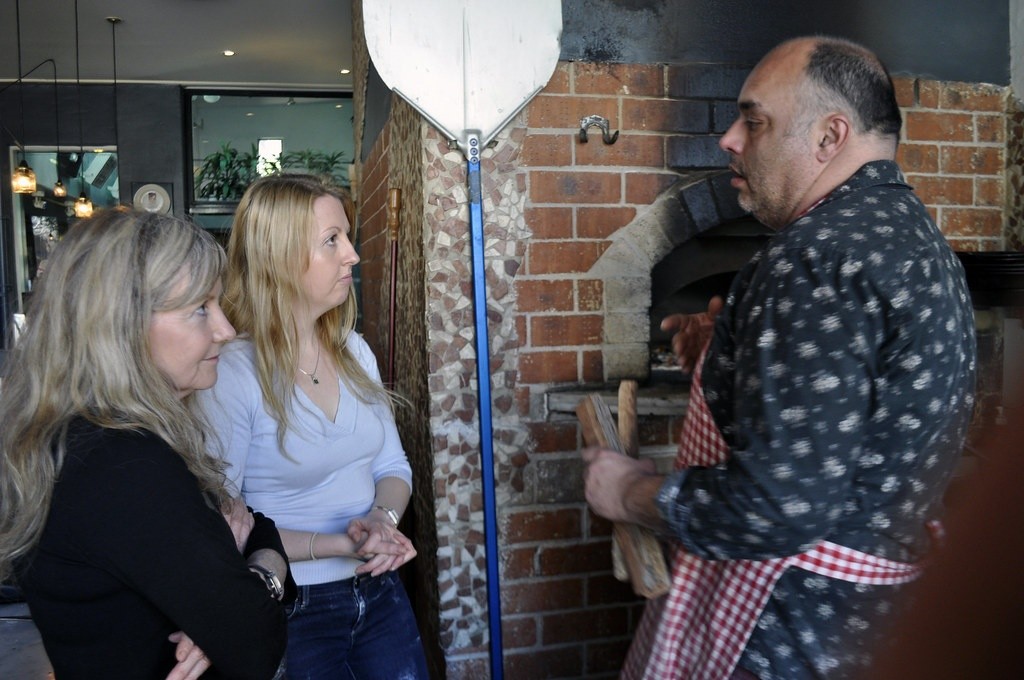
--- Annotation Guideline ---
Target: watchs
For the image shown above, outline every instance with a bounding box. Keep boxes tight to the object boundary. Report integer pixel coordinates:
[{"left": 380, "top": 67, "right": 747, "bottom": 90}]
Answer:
[
  {"left": 370, "top": 505, "right": 400, "bottom": 526},
  {"left": 249, "top": 563, "right": 283, "bottom": 599}
]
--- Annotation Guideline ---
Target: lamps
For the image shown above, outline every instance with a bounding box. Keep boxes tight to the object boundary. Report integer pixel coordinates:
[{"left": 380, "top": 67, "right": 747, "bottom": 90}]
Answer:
[
  {"left": 71, "top": 0, "right": 95, "bottom": 219},
  {"left": 0, "top": 58, "right": 67, "bottom": 198}
]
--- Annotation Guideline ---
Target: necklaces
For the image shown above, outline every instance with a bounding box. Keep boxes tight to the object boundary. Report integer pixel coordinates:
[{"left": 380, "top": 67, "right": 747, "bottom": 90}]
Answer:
[{"left": 298, "top": 325, "right": 320, "bottom": 385}]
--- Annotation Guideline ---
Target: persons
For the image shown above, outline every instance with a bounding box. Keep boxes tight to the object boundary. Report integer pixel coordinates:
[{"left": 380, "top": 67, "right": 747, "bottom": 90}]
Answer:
[
  {"left": 0, "top": 205, "right": 299, "bottom": 680},
  {"left": 187, "top": 174, "right": 430, "bottom": 680},
  {"left": 578, "top": 34, "right": 977, "bottom": 680}
]
